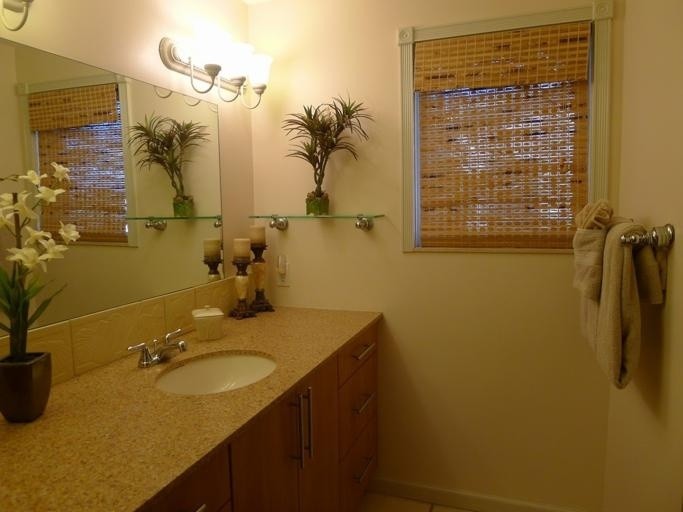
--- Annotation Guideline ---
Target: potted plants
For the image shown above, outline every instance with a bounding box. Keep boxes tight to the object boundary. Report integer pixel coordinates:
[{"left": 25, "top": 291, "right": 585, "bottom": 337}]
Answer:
[
  {"left": 281, "top": 88, "right": 376, "bottom": 215},
  {"left": 125, "top": 109, "right": 212, "bottom": 217}
]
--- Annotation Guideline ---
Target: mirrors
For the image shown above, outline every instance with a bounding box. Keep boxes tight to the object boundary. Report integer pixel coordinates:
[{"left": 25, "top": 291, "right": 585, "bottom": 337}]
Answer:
[{"left": 1, "top": 35, "right": 226, "bottom": 336}]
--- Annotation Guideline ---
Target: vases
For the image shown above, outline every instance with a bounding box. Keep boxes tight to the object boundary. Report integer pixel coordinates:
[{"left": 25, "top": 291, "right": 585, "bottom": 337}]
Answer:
[{"left": 1, "top": 355, "right": 54, "bottom": 424}]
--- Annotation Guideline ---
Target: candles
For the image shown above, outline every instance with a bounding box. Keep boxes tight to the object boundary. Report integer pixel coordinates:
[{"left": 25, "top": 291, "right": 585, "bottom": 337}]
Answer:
[
  {"left": 246, "top": 225, "right": 265, "bottom": 245},
  {"left": 232, "top": 237, "right": 251, "bottom": 262},
  {"left": 204, "top": 235, "right": 221, "bottom": 261}
]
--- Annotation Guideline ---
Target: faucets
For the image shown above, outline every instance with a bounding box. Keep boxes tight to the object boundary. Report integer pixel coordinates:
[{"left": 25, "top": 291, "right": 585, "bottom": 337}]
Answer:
[{"left": 152, "top": 340, "right": 185, "bottom": 368}]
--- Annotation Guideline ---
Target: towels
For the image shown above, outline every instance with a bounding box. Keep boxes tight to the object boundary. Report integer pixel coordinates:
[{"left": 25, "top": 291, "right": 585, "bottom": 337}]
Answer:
[{"left": 570, "top": 199, "right": 668, "bottom": 390}]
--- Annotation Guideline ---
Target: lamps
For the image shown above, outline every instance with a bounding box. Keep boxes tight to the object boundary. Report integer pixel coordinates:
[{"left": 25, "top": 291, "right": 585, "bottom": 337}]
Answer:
[{"left": 159, "top": 35, "right": 274, "bottom": 110}]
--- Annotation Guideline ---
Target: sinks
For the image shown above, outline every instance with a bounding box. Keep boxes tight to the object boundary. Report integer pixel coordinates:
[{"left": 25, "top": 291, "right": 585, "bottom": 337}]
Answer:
[{"left": 154, "top": 349, "right": 277, "bottom": 395}]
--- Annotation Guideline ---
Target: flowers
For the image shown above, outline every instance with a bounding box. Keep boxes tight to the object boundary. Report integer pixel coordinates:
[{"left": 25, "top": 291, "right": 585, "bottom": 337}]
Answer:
[{"left": 1, "top": 160, "right": 81, "bottom": 355}]
